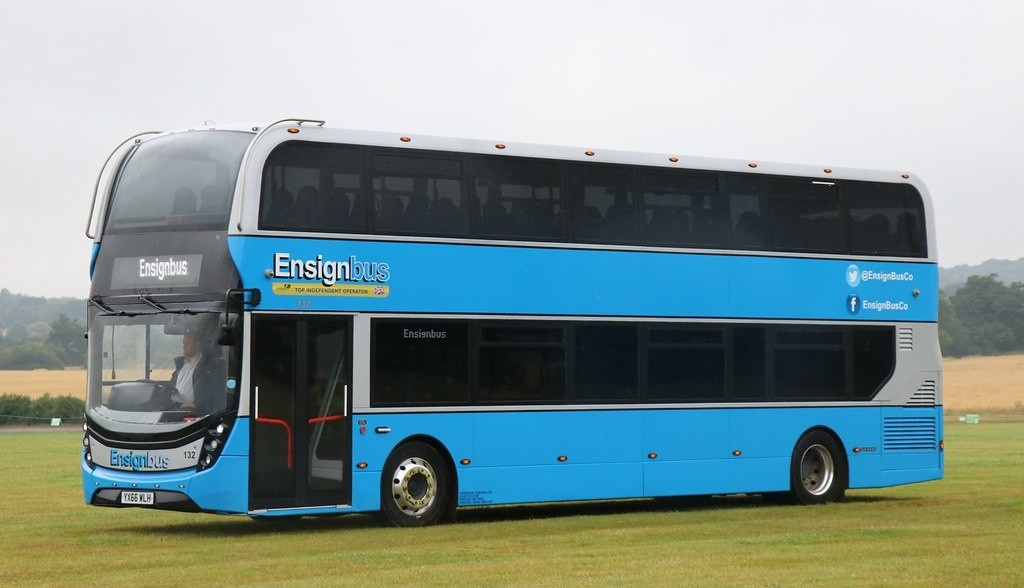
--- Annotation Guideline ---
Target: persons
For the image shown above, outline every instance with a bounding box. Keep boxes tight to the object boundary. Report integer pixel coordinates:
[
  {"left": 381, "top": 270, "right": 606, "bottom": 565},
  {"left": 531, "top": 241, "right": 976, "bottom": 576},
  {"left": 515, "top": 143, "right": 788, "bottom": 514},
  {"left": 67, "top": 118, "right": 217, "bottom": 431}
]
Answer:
[{"left": 161, "top": 332, "right": 215, "bottom": 409}]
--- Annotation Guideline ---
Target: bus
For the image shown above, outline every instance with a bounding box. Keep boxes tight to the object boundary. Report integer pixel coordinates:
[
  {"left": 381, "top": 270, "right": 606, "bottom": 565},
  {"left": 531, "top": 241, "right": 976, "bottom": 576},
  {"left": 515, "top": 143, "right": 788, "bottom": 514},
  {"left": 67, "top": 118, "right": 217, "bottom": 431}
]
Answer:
[{"left": 81, "top": 119, "right": 947, "bottom": 528}]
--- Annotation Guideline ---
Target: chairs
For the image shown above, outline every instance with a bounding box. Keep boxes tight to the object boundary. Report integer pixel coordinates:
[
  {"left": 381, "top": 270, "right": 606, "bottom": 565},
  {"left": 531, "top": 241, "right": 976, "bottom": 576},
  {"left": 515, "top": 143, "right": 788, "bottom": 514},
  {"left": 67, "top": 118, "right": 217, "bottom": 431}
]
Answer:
[
  {"left": 167, "top": 183, "right": 925, "bottom": 253},
  {"left": 173, "top": 355, "right": 215, "bottom": 410}
]
